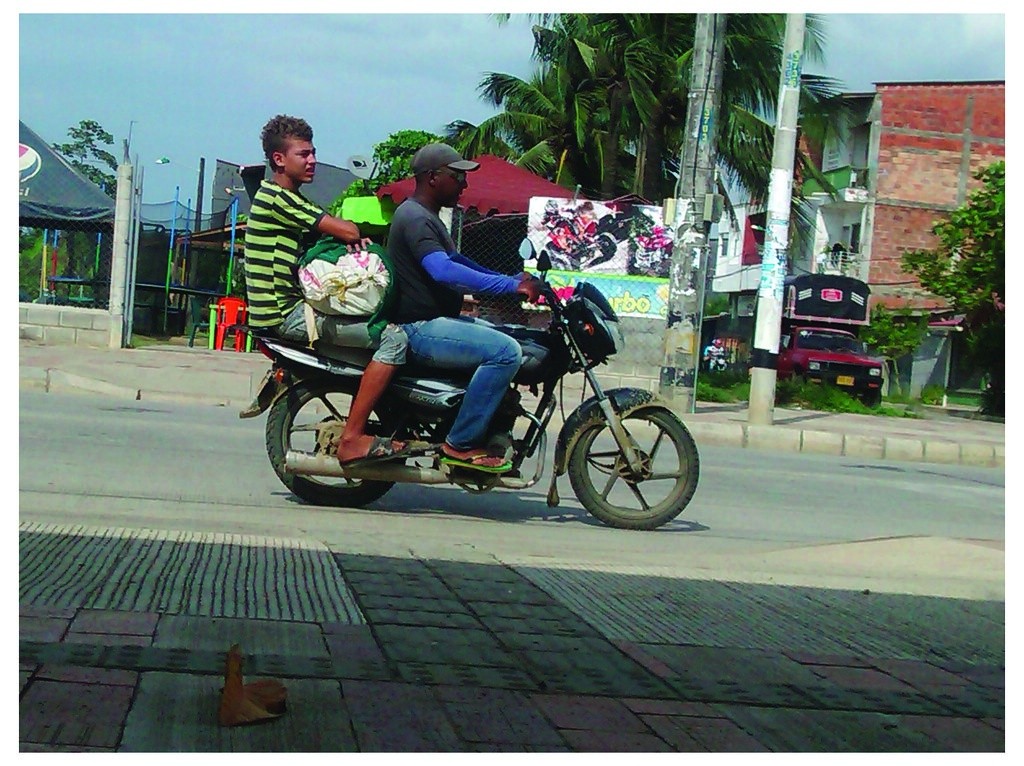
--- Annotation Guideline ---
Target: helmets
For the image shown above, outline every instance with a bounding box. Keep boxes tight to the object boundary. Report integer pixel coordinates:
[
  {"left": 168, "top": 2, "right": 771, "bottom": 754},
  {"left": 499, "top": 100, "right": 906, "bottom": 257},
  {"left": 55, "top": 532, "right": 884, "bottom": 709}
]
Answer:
[
  {"left": 545, "top": 201, "right": 560, "bottom": 214},
  {"left": 714, "top": 340, "right": 722, "bottom": 348}
]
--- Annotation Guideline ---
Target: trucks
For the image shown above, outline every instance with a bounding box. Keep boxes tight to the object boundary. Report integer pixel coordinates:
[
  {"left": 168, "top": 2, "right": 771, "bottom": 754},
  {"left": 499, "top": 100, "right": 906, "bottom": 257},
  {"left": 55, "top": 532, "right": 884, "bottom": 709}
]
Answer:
[{"left": 747, "top": 274, "right": 885, "bottom": 413}]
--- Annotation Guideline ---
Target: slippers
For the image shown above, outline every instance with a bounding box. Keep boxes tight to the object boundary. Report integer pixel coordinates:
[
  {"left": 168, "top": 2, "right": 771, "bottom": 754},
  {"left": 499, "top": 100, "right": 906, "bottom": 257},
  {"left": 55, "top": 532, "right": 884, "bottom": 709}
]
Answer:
[
  {"left": 440, "top": 454, "right": 513, "bottom": 473},
  {"left": 338, "top": 435, "right": 412, "bottom": 470}
]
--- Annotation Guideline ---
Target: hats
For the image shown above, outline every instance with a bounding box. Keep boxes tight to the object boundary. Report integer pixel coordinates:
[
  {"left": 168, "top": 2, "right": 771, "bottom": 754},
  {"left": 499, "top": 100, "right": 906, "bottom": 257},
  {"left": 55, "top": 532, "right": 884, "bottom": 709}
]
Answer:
[{"left": 410, "top": 144, "right": 480, "bottom": 175}]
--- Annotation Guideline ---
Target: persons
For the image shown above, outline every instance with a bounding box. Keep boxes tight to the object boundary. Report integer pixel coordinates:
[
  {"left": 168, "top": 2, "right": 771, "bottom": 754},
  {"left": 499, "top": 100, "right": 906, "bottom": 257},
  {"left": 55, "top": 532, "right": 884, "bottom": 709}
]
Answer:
[
  {"left": 703, "top": 339, "right": 724, "bottom": 370},
  {"left": 247, "top": 114, "right": 412, "bottom": 469},
  {"left": 386, "top": 143, "right": 543, "bottom": 473}
]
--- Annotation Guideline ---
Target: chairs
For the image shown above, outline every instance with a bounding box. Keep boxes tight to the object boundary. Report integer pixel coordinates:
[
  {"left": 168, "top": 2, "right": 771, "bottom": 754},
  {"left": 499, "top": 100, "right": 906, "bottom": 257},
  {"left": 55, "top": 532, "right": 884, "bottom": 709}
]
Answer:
[
  {"left": 215, "top": 297, "right": 246, "bottom": 351},
  {"left": 188, "top": 296, "right": 210, "bottom": 347}
]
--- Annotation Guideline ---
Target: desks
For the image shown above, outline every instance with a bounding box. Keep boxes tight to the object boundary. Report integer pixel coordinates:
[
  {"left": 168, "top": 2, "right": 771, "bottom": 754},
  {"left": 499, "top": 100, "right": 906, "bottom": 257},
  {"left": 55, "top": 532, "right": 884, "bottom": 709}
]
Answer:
[{"left": 208, "top": 303, "right": 253, "bottom": 352}]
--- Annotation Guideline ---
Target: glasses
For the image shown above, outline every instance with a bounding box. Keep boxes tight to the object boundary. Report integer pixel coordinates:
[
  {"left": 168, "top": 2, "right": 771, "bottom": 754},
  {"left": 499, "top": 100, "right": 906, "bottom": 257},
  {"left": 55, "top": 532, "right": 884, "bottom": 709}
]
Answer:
[{"left": 429, "top": 169, "right": 467, "bottom": 183}]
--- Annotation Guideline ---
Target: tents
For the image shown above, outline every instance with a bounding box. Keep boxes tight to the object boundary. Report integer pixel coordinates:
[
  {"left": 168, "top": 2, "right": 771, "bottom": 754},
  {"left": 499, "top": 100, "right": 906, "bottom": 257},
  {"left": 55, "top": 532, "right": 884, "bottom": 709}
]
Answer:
[
  {"left": 19, "top": 120, "right": 115, "bottom": 227},
  {"left": 377, "top": 154, "right": 588, "bottom": 215}
]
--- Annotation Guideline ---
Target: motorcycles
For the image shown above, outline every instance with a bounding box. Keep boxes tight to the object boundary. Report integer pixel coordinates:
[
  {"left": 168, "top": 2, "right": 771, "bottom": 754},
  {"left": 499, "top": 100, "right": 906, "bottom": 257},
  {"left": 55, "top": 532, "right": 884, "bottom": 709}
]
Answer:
[
  {"left": 238, "top": 236, "right": 700, "bottom": 531},
  {"left": 704, "top": 351, "right": 730, "bottom": 371}
]
